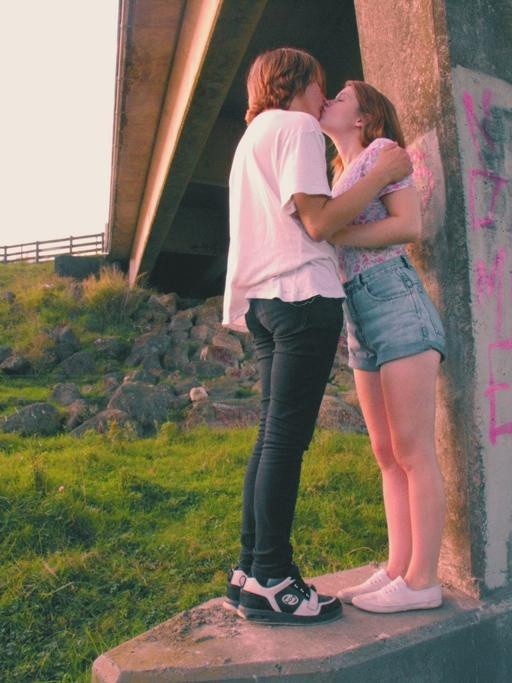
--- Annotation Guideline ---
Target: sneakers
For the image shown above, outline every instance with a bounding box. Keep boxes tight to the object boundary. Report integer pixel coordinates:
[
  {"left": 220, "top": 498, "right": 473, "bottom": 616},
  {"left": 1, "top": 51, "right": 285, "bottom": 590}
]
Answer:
[{"left": 222, "top": 566, "right": 443, "bottom": 625}]
